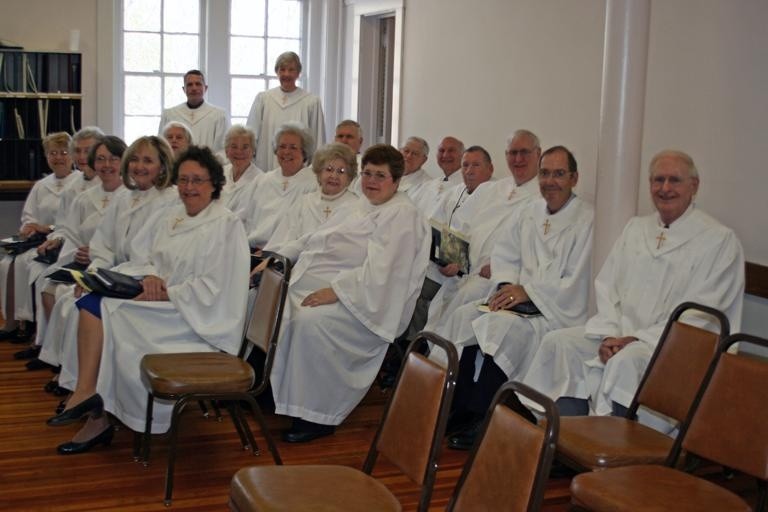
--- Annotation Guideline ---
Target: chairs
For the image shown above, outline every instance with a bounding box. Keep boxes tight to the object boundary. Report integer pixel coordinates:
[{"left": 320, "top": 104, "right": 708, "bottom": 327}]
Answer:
[{"left": 137, "top": 251, "right": 291, "bottom": 507}]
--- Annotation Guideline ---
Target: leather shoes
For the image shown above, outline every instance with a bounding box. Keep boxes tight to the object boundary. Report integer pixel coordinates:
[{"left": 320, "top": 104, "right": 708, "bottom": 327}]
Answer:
[
  {"left": 448, "top": 426, "right": 478, "bottom": 451},
  {"left": 0, "top": 322, "right": 114, "bottom": 455},
  {"left": 281, "top": 423, "right": 334, "bottom": 443}
]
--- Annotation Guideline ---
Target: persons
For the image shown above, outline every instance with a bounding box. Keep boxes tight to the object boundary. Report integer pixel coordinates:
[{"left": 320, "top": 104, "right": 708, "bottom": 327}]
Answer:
[
  {"left": 1, "top": 120, "right": 428, "bottom": 458},
  {"left": 242, "top": 51, "right": 324, "bottom": 172},
  {"left": 544, "top": 151, "right": 745, "bottom": 477},
  {"left": 156, "top": 69, "right": 230, "bottom": 166},
  {"left": 397, "top": 130, "right": 539, "bottom": 420},
  {"left": 445, "top": 145, "right": 595, "bottom": 449}
]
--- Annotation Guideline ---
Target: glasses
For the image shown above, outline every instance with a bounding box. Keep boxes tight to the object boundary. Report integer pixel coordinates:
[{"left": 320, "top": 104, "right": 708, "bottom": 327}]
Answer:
[
  {"left": 276, "top": 143, "right": 303, "bottom": 153},
  {"left": 537, "top": 169, "right": 570, "bottom": 179},
  {"left": 94, "top": 156, "right": 121, "bottom": 165},
  {"left": 506, "top": 148, "right": 537, "bottom": 158},
  {"left": 359, "top": 171, "right": 394, "bottom": 182},
  {"left": 47, "top": 151, "right": 71, "bottom": 158},
  {"left": 175, "top": 177, "right": 211, "bottom": 185},
  {"left": 321, "top": 166, "right": 347, "bottom": 176}
]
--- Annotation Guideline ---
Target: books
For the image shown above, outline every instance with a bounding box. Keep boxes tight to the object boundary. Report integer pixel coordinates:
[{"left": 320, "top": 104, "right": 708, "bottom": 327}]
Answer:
[
  {"left": 1, "top": 98, "right": 81, "bottom": 181},
  {"left": 0, "top": 52, "right": 82, "bottom": 95}
]
